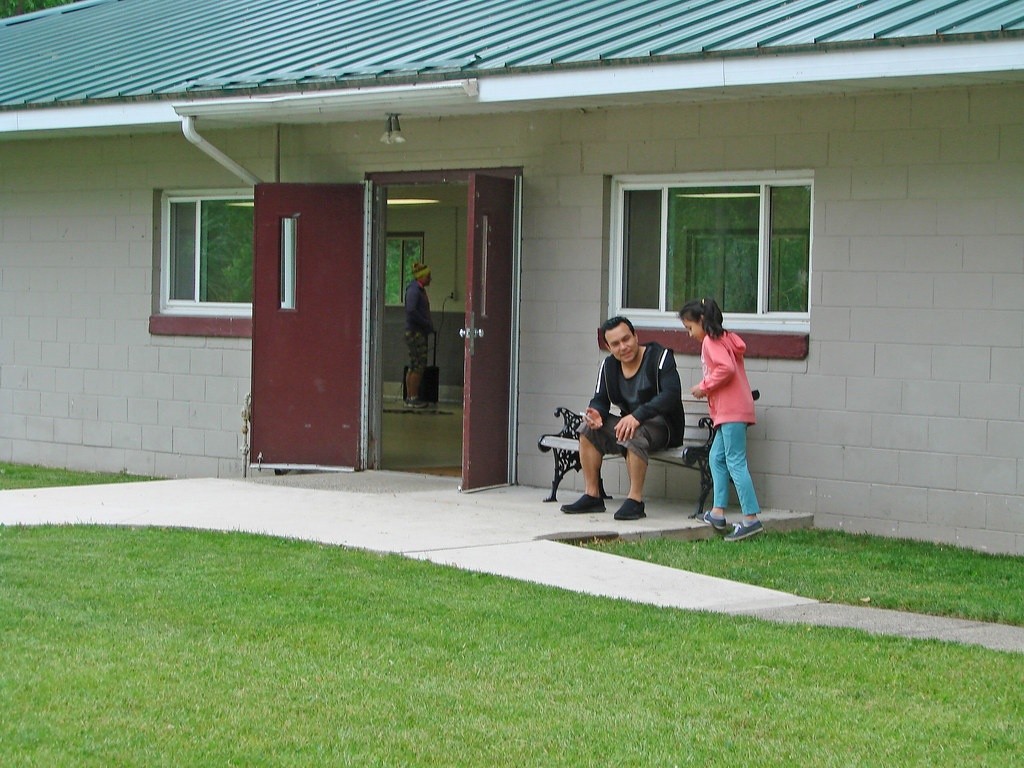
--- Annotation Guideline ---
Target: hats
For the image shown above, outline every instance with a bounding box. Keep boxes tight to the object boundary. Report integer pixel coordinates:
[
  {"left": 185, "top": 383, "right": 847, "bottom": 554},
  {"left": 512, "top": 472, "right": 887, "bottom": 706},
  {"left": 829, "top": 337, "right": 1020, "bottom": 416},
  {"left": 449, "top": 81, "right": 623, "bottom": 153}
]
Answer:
[{"left": 412, "top": 262, "right": 430, "bottom": 279}]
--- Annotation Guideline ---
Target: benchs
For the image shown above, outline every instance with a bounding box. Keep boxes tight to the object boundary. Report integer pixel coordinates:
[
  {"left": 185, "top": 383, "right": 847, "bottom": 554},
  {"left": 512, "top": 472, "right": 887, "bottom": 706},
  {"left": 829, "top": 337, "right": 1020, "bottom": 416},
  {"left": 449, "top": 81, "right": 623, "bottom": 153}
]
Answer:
[{"left": 537, "top": 382, "right": 761, "bottom": 519}]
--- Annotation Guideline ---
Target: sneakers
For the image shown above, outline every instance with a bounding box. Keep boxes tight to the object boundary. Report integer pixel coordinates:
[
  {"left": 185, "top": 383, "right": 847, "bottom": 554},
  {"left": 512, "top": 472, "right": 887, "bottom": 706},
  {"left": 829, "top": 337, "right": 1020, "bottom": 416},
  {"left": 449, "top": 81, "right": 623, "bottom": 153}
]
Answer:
[
  {"left": 614, "top": 498, "right": 646, "bottom": 519},
  {"left": 695, "top": 511, "right": 726, "bottom": 529},
  {"left": 723, "top": 520, "right": 764, "bottom": 541},
  {"left": 560, "top": 494, "right": 606, "bottom": 514}
]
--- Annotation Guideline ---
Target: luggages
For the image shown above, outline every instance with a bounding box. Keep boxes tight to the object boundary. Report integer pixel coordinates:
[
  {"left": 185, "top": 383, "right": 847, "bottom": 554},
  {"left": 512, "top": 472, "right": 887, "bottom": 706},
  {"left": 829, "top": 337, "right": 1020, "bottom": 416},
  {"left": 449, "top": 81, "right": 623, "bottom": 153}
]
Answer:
[{"left": 402, "top": 330, "right": 439, "bottom": 407}]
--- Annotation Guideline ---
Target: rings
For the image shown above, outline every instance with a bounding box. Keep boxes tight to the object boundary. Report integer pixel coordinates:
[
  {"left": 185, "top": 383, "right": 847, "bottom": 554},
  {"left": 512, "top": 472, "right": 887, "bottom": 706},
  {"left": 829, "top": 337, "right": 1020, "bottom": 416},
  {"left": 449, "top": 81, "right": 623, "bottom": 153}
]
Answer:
[{"left": 627, "top": 427, "right": 630, "bottom": 430}]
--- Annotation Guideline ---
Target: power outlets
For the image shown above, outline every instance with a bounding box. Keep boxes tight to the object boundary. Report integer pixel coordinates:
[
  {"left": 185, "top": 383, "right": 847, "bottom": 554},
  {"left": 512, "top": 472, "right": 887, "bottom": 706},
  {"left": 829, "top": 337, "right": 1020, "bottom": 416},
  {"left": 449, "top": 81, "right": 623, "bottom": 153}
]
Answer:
[{"left": 450, "top": 291, "right": 458, "bottom": 301}]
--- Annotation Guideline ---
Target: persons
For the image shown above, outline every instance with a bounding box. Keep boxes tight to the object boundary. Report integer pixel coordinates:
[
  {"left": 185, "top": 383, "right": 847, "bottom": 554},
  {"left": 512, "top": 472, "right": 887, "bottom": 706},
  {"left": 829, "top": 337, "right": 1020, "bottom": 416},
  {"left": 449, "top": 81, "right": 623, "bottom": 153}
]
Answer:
[
  {"left": 404, "top": 262, "right": 432, "bottom": 408},
  {"left": 679, "top": 297, "right": 764, "bottom": 541},
  {"left": 560, "top": 316, "right": 685, "bottom": 520}
]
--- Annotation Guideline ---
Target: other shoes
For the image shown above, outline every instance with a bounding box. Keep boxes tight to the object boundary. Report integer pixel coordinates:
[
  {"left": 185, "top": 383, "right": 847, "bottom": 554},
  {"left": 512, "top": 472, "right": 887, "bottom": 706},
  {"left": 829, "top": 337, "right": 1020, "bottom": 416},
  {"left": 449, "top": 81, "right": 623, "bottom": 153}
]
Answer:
[{"left": 404, "top": 397, "right": 427, "bottom": 408}]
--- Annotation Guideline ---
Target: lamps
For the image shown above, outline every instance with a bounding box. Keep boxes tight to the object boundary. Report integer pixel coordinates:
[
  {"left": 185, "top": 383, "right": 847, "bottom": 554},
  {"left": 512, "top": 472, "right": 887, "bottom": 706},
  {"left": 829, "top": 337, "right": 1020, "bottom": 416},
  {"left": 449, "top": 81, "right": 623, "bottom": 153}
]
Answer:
[{"left": 381, "top": 113, "right": 407, "bottom": 144}]
